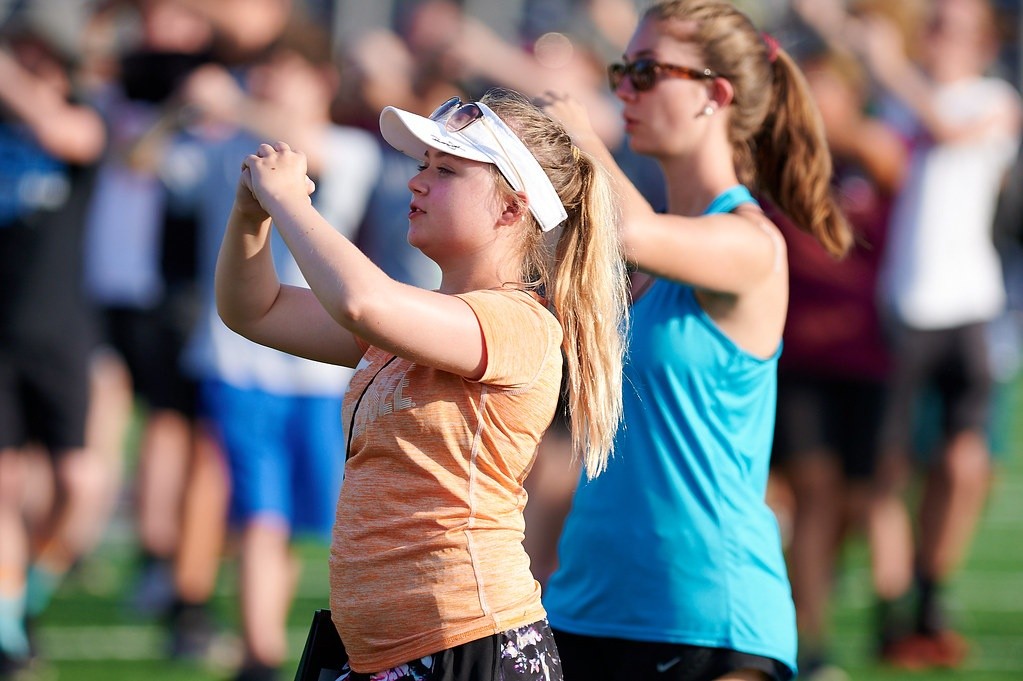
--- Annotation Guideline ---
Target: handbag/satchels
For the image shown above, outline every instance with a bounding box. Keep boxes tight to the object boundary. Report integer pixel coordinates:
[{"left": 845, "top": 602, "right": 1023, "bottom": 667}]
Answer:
[{"left": 294, "top": 609, "right": 348, "bottom": 681}]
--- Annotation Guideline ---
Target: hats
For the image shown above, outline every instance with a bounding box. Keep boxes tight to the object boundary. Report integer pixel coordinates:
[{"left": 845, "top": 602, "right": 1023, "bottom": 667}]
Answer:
[{"left": 378, "top": 102, "right": 569, "bottom": 232}]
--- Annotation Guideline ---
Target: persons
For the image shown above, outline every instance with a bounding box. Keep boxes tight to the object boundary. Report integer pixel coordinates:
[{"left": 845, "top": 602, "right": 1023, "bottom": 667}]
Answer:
[
  {"left": 0, "top": 0, "right": 1023, "bottom": 681},
  {"left": 531, "top": 0, "right": 852, "bottom": 681},
  {"left": 214, "top": 86, "right": 624, "bottom": 681}
]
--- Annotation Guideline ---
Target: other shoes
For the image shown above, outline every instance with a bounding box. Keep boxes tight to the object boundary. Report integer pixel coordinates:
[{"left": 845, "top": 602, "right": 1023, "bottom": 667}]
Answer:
[{"left": 886, "top": 632, "right": 972, "bottom": 673}]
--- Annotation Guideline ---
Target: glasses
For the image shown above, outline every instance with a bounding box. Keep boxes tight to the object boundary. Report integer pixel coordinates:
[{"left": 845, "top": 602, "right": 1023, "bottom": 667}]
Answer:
[
  {"left": 606, "top": 58, "right": 737, "bottom": 103},
  {"left": 428, "top": 95, "right": 525, "bottom": 190}
]
